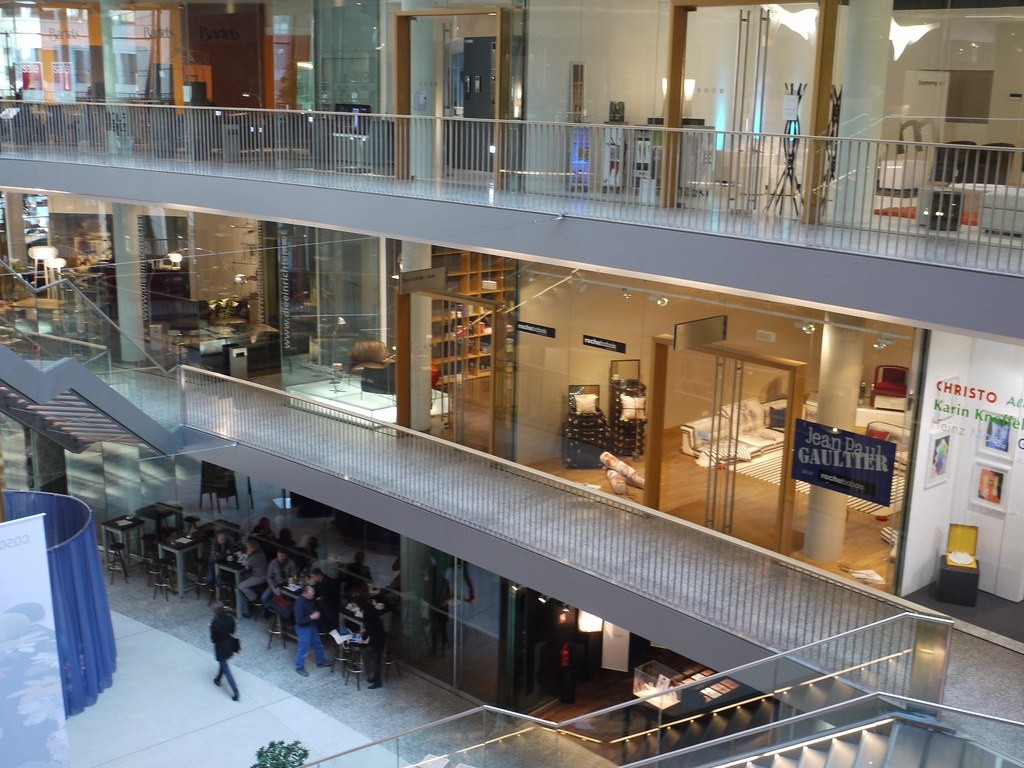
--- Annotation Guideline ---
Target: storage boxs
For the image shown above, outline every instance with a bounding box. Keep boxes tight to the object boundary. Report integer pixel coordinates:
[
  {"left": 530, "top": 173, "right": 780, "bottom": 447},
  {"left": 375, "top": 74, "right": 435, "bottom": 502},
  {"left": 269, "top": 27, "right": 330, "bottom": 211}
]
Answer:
[{"left": 632, "top": 661, "right": 684, "bottom": 710}]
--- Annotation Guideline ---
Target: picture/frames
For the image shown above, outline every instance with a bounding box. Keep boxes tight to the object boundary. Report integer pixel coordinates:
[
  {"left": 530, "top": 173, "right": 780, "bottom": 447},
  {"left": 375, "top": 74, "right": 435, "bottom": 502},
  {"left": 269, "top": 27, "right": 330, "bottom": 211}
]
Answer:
[
  {"left": 923, "top": 430, "right": 954, "bottom": 489},
  {"left": 969, "top": 457, "right": 1012, "bottom": 514},
  {"left": 976, "top": 408, "right": 1020, "bottom": 462},
  {"left": 936, "top": 376, "right": 961, "bottom": 422}
]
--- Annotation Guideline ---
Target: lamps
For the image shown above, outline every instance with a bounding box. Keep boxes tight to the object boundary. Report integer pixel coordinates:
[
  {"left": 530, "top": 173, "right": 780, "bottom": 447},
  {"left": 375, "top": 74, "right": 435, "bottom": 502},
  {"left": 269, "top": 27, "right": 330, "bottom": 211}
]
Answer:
[
  {"left": 684, "top": 79, "right": 696, "bottom": 117},
  {"left": 240, "top": 91, "right": 263, "bottom": 125},
  {"left": 660, "top": 78, "right": 667, "bottom": 116},
  {"left": 331, "top": 317, "right": 347, "bottom": 364}
]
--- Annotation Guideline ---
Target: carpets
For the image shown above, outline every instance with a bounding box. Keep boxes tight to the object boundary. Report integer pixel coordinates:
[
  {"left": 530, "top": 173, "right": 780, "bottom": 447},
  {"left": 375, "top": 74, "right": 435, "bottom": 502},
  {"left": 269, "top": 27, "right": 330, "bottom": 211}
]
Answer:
[
  {"left": 728, "top": 448, "right": 905, "bottom": 516},
  {"left": 875, "top": 179, "right": 918, "bottom": 199},
  {"left": 874, "top": 205, "right": 979, "bottom": 226}
]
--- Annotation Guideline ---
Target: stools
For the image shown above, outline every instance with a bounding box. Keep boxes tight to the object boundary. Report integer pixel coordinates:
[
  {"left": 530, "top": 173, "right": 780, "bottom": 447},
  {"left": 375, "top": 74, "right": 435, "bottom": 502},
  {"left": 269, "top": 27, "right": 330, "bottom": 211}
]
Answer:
[
  {"left": 331, "top": 639, "right": 349, "bottom": 678},
  {"left": 193, "top": 558, "right": 207, "bottom": 600},
  {"left": 184, "top": 514, "right": 200, "bottom": 532},
  {"left": 268, "top": 608, "right": 289, "bottom": 650},
  {"left": 140, "top": 534, "right": 157, "bottom": 571},
  {"left": 345, "top": 643, "right": 371, "bottom": 692},
  {"left": 108, "top": 543, "right": 129, "bottom": 585},
  {"left": 214, "top": 584, "right": 236, "bottom": 617},
  {"left": 382, "top": 632, "right": 401, "bottom": 682},
  {"left": 147, "top": 548, "right": 175, "bottom": 587},
  {"left": 248, "top": 582, "right": 265, "bottom": 621},
  {"left": 152, "top": 559, "right": 176, "bottom": 601}
]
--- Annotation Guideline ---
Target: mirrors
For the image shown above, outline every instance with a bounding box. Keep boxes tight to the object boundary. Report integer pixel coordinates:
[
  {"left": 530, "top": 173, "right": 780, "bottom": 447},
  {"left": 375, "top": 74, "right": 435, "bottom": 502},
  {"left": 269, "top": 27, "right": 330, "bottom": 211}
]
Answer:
[
  {"left": 611, "top": 360, "right": 640, "bottom": 381},
  {"left": 568, "top": 384, "right": 600, "bottom": 407},
  {"left": 569, "top": 61, "right": 586, "bottom": 123}
]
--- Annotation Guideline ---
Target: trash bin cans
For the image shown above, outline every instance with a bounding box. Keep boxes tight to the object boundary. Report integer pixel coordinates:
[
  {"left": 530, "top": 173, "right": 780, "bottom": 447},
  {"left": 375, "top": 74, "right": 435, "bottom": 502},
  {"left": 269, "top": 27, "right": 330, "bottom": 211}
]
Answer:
[
  {"left": 222, "top": 125, "right": 242, "bottom": 164},
  {"left": 229, "top": 346, "right": 248, "bottom": 382},
  {"left": 149, "top": 323, "right": 162, "bottom": 350}
]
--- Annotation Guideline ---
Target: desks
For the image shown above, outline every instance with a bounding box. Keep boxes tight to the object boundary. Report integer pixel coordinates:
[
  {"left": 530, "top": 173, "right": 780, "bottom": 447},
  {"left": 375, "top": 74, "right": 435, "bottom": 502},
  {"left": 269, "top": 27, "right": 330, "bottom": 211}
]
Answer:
[
  {"left": 99, "top": 514, "right": 145, "bottom": 570},
  {"left": 197, "top": 519, "right": 241, "bottom": 564},
  {"left": 213, "top": 559, "right": 247, "bottom": 620},
  {"left": 332, "top": 132, "right": 372, "bottom": 170},
  {"left": 76, "top": 96, "right": 161, "bottom": 146},
  {"left": 157, "top": 531, "right": 205, "bottom": 599},
  {"left": 134, "top": 503, "right": 184, "bottom": 554},
  {"left": 276, "top": 582, "right": 316, "bottom": 641},
  {"left": 7, "top": 298, "right": 70, "bottom": 356},
  {"left": 336, "top": 606, "right": 392, "bottom": 662}
]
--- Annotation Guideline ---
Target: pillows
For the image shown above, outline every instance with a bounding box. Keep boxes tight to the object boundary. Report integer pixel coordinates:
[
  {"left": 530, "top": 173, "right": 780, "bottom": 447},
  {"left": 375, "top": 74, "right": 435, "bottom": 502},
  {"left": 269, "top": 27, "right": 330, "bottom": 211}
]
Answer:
[
  {"left": 574, "top": 394, "right": 598, "bottom": 414},
  {"left": 768, "top": 406, "right": 786, "bottom": 429},
  {"left": 619, "top": 396, "right": 647, "bottom": 421},
  {"left": 866, "top": 428, "right": 891, "bottom": 441},
  {"left": 230, "top": 334, "right": 252, "bottom": 345},
  {"left": 256, "top": 331, "right": 279, "bottom": 341}
]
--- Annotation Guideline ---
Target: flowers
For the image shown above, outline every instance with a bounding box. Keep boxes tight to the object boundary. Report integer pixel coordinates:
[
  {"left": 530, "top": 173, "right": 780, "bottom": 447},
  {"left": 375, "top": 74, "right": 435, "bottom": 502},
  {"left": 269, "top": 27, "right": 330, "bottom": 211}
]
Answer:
[{"left": 207, "top": 298, "right": 240, "bottom": 327}]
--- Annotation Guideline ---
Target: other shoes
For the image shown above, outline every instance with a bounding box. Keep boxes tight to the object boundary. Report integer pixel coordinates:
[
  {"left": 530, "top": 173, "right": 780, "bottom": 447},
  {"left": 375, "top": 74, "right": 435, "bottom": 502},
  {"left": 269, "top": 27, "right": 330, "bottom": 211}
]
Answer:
[
  {"left": 232, "top": 694, "right": 239, "bottom": 701},
  {"left": 214, "top": 679, "right": 221, "bottom": 686},
  {"left": 316, "top": 660, "right": 334, "bottom": 668},
  {"left": 295, "top": 666, "right": 309, "bottom": 677},
  {"left": 368, "top": 670, "right": 382, "bottom": 689}
]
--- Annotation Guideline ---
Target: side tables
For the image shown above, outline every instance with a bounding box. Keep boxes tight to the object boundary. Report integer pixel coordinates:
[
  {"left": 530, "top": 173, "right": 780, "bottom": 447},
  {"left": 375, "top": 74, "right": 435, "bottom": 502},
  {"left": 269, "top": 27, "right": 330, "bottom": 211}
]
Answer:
[{"left": 171, "top": 341, "right": 187, "bottom": 367}]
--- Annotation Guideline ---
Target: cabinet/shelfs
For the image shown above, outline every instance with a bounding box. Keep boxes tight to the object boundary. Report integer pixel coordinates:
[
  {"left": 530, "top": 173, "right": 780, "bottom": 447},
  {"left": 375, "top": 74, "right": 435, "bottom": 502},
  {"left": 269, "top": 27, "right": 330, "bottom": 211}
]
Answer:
[
  {"left": 608, "top": 380, "right": 647, "bottom": 457},
  {"left": 0, "top": 194, "right": 49, "bottom": 243},
  {"left": 564, "top": 408, "right": 607, "bottom": 470},
  {"left": 392, "top": 239, "right": 515, "bottom": 388},
  {"left": 560, "top": 122, "right": 593, "bottom": 193},
  {"left": 445, "top": 35, "right": 523, "bottom": 172}
]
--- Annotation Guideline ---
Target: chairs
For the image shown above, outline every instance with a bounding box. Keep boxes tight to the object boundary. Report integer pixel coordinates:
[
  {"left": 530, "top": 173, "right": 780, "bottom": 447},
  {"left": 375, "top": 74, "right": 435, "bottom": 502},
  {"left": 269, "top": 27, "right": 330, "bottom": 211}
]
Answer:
[
  {"left": 63, "top": 310, "right": 92, "bottom": 356},
  {"left": 346, "top": 340, "right": 389, "bottom": 375},
  {"left": 359, "top": 363, "right": 397, "bottom": 401},
  {"left": 3, "top": 306, "right": 38, "bottom": 354}
]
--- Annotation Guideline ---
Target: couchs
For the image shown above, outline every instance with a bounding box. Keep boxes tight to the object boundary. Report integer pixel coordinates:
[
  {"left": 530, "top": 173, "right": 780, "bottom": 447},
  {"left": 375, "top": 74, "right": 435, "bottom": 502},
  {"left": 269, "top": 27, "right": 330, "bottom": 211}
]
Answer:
[
  {"left": 879, "top": 158, "right": 932, "bottom": 193},
  {"left": 95, "top": 300, "right": 210, "bottom": 336},
  {"left": 865, "top": 421, "right": 911, "bottom": 471},
  {"left": 680, "top": 415, "right": 760, "bottom": 467},
  {"left": 949, "top": 182, "right": 1018, "bottom": 215},
  {"left": 965, "top": 142, "right": 1015, "bottom": 185},
  {"left": 869, "top": 365, "right": 909, "bottom": 412},
  {"left": 716, "top": 397, "right": 785, "bottom": 455},
  {"left": 762, "top": 399, "right": 816, "bottom": 433},
  {"left": 978, "top": 188, "right": 1024, "bottom": 238},
  {"left": 934, "top": 140, "right": 976, "bottom": 183},
  {"left": 714, "top": 150, "right": 803, "bottom": 190},
  {"left": 208, "top": 306, "right": 249, "bottom": 325},
  {"left": 184, "top": 334, "right": 311, "bottom": 375}
]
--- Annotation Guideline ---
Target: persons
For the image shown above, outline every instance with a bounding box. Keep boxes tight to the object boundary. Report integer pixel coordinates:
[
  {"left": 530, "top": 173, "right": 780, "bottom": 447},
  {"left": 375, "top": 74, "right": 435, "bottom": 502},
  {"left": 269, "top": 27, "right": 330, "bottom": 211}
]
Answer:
[
  {"left": 310, "top": 567, "right": 348, "bottom": 629},
  {"left": 261, "top": 547, "right": 298, "bottom": 620},
  {"left": 351, "top": 583, "right": 386, "bottom": 689},
  {"left": 209, "top": 601, "right": 241, "bottom": 702},
  {"left": 207, "top": 534, "right": 231, "bottom": 592},
  {"left": 251, "top": 517, "right": 475, "bottom": 659},
  {"left": 293, "top": 585, "right": 334, "bottom": 677},
  {"left": 238, "top": 540, "right": 268, "bottom": 606}
]
